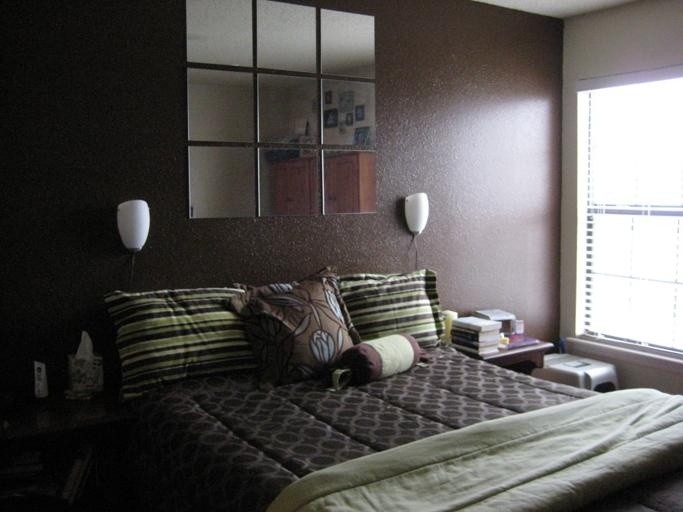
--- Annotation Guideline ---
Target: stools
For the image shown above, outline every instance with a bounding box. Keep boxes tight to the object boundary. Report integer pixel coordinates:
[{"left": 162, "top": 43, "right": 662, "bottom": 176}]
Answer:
[{"left": 531, "top": 352, "right": 620, "bottom": 392}]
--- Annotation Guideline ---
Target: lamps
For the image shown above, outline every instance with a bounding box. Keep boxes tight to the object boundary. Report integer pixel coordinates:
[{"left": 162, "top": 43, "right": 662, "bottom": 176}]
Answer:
[
  {"left": 402, "top": 193, "right": 429, "bottom": 272},
  {"left": 115, "top": 199, "right": 151, "bottom": 294}
]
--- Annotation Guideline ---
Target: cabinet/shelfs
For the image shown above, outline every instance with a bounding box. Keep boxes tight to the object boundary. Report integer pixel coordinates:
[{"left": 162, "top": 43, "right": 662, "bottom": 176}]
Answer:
[{"left": 267, "top": 149, "right": 374, "bottom": 213}]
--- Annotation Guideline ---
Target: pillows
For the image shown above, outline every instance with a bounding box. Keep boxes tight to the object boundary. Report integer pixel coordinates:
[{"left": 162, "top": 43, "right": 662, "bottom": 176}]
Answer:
[
  {"left": 228, "top": 265, "right": 359, "bottom": 384},
  {"left": 332, "top": 269, "right": 449, "bottom": 351},
  {"left": 104, "top": 285, "right": 257, "bottom": 400}
]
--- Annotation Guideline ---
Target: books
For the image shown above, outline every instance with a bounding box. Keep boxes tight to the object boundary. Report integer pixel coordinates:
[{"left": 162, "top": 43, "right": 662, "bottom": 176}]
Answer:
[{"left": 450, "top": 304, "right": 518, "bottom": 358}]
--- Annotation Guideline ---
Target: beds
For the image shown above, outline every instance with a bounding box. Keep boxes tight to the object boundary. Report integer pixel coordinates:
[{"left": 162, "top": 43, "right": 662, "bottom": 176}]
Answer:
[{"left": 98, "top": 342, "right": 682, "bottom": 511}]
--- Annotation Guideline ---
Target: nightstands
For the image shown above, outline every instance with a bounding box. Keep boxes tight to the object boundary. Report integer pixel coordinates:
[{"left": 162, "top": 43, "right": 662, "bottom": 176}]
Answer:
[
  {"left": 451, "top": 331, "right": 555, "bottom": 370},
  {"left": 0, "top": 391, "right": 141, "bottom": 511}
]
